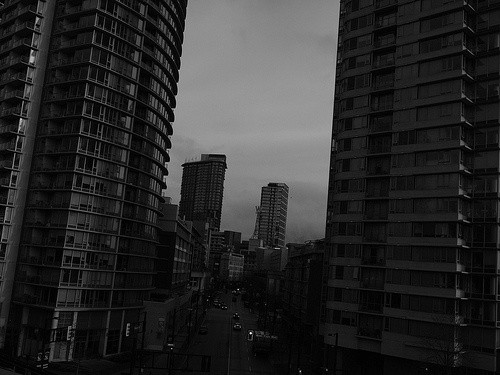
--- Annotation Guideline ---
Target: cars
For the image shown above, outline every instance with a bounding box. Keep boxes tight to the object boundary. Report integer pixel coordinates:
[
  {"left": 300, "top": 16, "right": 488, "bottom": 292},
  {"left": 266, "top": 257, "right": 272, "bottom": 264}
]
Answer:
[
  {"left": 231, "top": 312, "right": 240, "bottom": 330},
  {"left": 231, "top": 286, "right": 241, "bottom": 296}
]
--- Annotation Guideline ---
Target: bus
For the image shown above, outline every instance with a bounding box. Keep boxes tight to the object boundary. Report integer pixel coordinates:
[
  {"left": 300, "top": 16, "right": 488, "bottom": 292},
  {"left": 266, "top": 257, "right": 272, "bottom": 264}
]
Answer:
[{"left": 253, "top": 329, "right": 271, "bottom": 355}]
